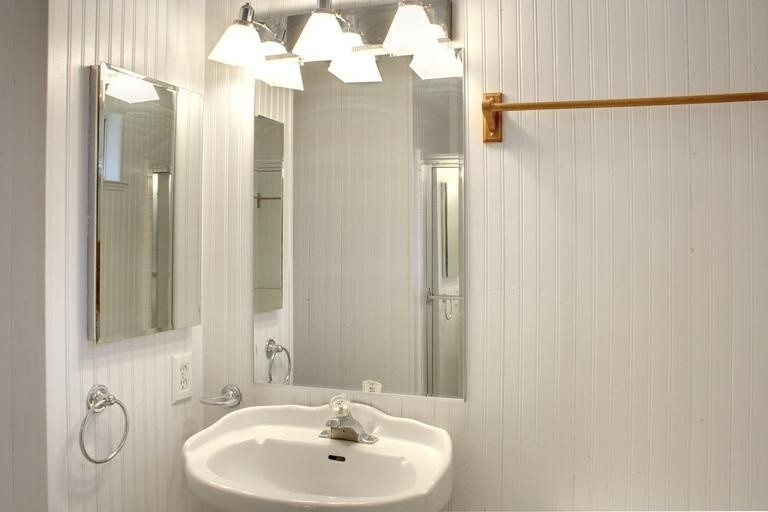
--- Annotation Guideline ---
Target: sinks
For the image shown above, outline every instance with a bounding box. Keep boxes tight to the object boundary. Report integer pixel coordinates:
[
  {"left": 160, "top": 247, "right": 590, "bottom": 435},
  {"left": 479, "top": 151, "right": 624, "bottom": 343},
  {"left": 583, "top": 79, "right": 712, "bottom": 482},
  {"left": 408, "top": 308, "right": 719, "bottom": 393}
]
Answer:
[{"left": 179, "top": 402, "right": 454, "bottom": 512}]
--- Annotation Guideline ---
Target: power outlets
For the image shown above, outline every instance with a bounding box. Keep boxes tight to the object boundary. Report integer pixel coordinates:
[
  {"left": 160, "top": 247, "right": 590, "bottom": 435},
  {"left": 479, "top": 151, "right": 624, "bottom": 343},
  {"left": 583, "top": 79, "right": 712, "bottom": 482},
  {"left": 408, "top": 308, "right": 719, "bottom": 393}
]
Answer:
[{"left": 171, "top": 351, "right": 193, "bottom": 405}]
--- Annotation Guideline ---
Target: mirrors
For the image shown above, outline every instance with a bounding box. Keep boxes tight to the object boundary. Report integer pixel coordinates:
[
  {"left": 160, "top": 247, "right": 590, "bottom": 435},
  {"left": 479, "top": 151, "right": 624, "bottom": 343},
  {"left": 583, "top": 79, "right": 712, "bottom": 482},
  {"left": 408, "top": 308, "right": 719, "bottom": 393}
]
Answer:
[
  {"left": 252, "top": 113, "right": 284, "bottom": 312},
  {"left": 251, "top": 0, "right": 466, "bottom": 398},
  {"left": 89, "top": 62, "right": 203, "bottom": 348}
]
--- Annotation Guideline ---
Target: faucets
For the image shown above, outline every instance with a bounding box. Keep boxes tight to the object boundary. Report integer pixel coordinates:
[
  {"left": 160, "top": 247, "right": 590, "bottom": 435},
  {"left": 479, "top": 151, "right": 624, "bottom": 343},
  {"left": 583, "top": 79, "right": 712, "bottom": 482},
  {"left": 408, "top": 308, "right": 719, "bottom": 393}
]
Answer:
[
  {"left": 361, "top": 379, "right": 381, "bottom": 393},
  {"left": 320, "top": 392, "right": 379, "bottom": 444}
]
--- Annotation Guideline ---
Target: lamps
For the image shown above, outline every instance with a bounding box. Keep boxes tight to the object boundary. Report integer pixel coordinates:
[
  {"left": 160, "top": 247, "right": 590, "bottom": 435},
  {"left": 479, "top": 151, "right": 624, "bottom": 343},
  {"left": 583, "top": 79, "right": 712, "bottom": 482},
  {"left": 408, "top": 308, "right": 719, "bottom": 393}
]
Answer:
[{"left": 206, "top": 0, "right": 465, "bottom": 91}]
